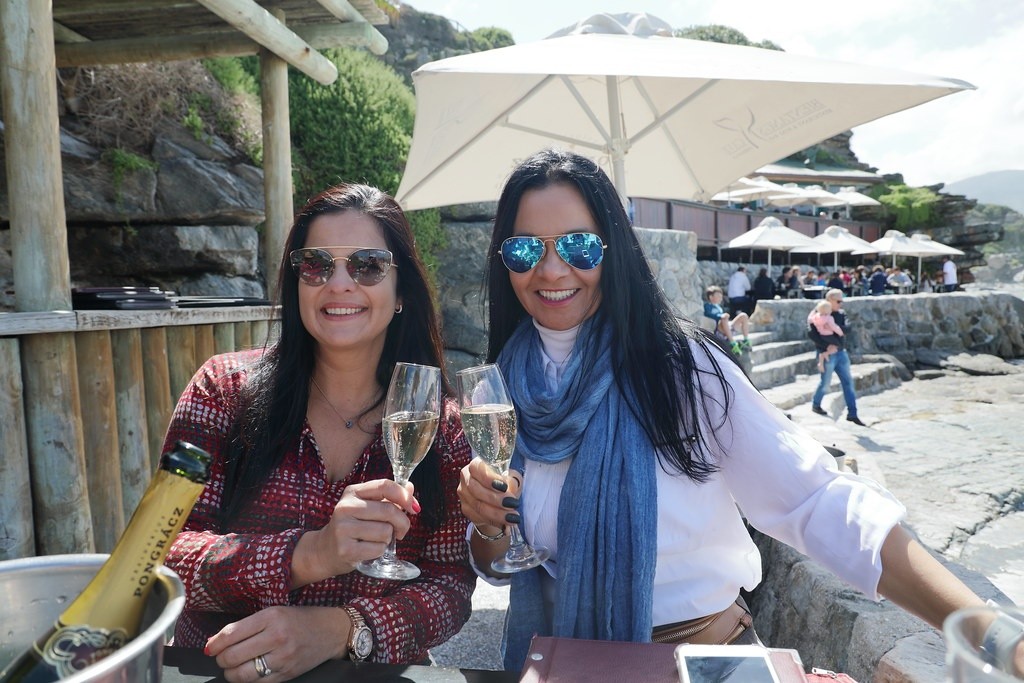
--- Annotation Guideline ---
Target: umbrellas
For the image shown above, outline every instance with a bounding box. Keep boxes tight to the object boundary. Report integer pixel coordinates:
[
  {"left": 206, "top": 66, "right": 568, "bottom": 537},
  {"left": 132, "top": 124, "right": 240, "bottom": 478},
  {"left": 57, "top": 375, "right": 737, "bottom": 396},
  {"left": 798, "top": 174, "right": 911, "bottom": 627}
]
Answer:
[
  {"left": 721, "top": 216, "right": 965, "bottom": 283},
  {"left": 711, "top": 176, "right": 881, "bottom": 218},
  {"left": 393, "top": 35, "right": 979, "bottom": 220}
]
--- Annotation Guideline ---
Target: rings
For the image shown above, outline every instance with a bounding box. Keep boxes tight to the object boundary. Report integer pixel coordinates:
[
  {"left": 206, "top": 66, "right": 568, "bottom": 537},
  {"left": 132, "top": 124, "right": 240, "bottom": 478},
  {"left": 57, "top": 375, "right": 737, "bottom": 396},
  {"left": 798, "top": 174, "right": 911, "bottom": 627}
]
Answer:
[{"left": 255, "top": 656, "right": 271, "bottom": 676}]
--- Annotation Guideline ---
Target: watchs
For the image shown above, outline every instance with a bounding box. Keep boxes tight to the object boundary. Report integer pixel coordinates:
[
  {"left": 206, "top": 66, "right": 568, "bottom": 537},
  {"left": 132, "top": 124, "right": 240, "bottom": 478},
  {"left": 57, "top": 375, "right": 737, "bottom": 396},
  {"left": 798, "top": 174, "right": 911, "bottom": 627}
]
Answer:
[{"left": 341, "top": 604, "right": 373, "bottom": 662}]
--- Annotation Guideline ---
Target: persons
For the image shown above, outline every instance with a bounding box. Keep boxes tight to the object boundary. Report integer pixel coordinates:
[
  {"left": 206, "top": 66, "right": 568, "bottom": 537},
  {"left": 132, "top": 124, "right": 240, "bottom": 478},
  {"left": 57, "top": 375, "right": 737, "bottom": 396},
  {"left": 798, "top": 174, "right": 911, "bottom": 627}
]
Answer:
[
  {"left": 158, "top": 182, "right": 479, "bottom": 683},
  {"left": 728, "top": 256, "right": 958, "bottom": 320},
  {"left": 812, "top": 300, "right": 844, "bottom": 372},
  {"left": 743, "top": 205, "right": 840, "bottom": 220},
  {"left": 704, "top": 285, "right": 752, "bottom": 358},
  {"left": 457, "top": 149, "right": 1024, "bottom": 683},
  {"left": 807, "top": 289, "right": 864, "bottom": 426}
]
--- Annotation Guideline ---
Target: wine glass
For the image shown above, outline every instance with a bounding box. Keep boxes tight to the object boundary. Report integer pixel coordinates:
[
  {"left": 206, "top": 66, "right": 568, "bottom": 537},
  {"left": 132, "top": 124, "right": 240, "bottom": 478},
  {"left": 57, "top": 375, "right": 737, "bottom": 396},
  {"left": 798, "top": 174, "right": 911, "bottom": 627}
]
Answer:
[
  {"left": 457, "top": 364, "right": 551, "bottom": 573},
  {"left": 356, "top": 362, "right": 441, "bottom": 581}
]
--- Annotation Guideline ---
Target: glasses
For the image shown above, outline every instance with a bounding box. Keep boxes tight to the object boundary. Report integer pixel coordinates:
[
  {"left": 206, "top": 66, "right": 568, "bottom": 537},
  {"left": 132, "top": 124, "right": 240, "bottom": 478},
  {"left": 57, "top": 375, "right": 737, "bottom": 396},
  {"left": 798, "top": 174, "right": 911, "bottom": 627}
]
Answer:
[
  {"left": 290, "top": 246, "right": 398, "bottom": 287},
  {"left": 498, "top": 233, "right": 608, "bottom": 274},
  {"left": 833, "top": 299, "right": 843, "bottom": 304}
]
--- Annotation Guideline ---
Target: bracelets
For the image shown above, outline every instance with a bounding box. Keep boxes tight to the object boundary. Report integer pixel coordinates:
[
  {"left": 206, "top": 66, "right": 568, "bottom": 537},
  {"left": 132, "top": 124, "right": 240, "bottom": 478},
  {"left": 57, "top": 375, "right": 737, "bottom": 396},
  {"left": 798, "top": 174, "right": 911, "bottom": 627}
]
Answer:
[
  {"left": 980, "top": 612, "right": 1024, "bottom": 674},
  {"left": 473, "top": 524, "right": 505, "bottom": 541}
]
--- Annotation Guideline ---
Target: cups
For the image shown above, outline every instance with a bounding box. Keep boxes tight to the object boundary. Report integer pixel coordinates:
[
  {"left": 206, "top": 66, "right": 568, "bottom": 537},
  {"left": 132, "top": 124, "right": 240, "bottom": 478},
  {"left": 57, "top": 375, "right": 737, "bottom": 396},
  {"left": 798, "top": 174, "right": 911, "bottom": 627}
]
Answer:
[{"left": 944, "top": 605, "right": 1024, "bottom": 683}]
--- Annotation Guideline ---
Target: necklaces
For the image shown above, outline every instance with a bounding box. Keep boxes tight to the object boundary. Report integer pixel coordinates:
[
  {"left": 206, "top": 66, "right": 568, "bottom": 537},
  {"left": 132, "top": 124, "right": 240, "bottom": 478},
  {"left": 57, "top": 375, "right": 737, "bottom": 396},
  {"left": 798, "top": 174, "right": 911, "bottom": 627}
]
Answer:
[{"left": 312, "top": 377, "right": 381, "bottom": 428}]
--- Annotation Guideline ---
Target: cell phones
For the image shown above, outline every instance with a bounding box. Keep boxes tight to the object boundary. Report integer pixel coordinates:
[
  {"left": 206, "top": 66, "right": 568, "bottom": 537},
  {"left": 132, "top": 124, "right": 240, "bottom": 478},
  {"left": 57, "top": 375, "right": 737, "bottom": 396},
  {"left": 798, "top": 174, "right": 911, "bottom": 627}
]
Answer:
[{"left": 674, "top": 642, "right": 781, "bottom": 683}]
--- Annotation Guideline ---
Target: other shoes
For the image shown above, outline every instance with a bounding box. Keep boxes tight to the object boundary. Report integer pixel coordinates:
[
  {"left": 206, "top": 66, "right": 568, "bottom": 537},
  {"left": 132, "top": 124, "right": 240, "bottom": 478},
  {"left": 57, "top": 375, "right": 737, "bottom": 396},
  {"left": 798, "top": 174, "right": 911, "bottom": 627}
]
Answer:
[
  {"left": 812, "top": 407, "right": 827, "bottom": 414},
  {"left": 729, "top": 341, "right": 741, "bottom": 357},
  {"left": 739, "top": 339, "right": 752, "bottom": 351},
  {"left": 847, "top": 414, "right": 865, "bottom": 426}
]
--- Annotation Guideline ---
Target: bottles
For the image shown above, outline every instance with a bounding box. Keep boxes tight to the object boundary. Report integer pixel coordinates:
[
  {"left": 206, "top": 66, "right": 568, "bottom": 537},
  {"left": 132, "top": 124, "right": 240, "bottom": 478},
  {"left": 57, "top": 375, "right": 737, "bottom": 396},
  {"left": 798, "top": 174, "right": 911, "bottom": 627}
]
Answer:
[{"left": 0, "top": 439, "right": 213, "bottom": 682}]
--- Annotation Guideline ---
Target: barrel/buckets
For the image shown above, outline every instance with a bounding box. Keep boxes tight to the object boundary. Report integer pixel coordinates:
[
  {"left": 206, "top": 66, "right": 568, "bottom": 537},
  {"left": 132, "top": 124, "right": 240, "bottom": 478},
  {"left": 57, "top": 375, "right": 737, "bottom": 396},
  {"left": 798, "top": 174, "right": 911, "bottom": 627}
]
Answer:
[{"left": 0, "top": 554, "right": 186, "bottom": 683}]
